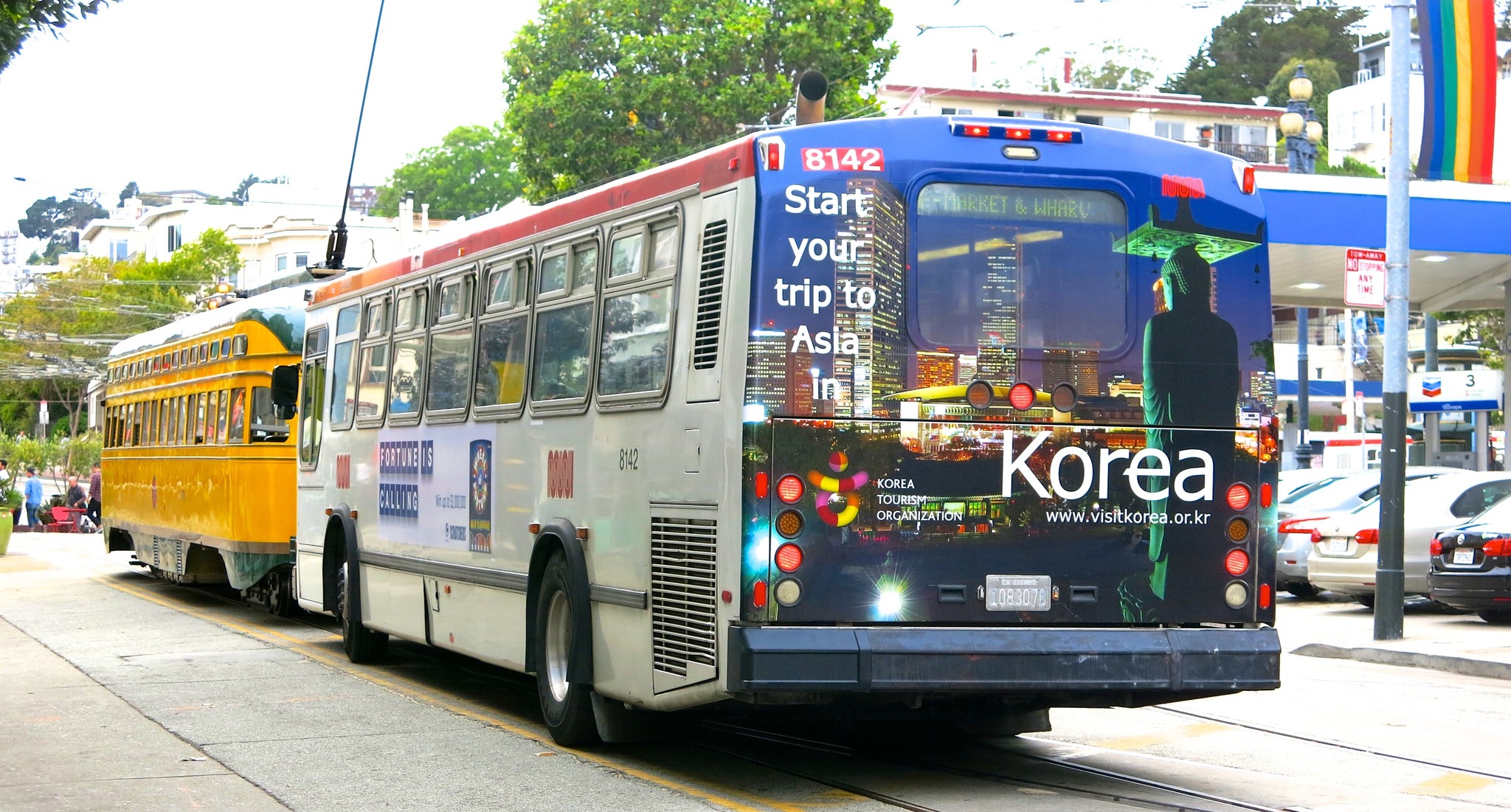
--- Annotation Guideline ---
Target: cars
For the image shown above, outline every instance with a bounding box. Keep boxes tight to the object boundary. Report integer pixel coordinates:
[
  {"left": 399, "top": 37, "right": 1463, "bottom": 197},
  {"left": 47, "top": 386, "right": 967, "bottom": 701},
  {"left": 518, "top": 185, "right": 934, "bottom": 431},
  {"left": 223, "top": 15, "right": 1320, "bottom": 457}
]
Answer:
[{"left": 1277, "top": 465, "right": 1511, "bottom": 626}]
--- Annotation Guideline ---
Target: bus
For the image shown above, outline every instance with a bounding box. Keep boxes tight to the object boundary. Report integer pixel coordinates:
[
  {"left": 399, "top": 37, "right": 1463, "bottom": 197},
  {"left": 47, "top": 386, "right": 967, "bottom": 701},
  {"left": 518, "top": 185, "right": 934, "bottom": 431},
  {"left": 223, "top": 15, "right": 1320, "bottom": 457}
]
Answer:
[
  {"left": 272, "top": 116, "right": 1283, "bottom": 749},
  {"left": 103, "top": 261, "right": 347, "bottom": 620}
]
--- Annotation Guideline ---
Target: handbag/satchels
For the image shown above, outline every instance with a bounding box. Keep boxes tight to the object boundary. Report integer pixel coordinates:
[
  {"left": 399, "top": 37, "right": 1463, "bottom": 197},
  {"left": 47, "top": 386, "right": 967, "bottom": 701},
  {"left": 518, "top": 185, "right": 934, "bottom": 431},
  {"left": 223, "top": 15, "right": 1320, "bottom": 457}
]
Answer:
[{"left": 80, "top": 500, "right": 88, "bottom": 516}]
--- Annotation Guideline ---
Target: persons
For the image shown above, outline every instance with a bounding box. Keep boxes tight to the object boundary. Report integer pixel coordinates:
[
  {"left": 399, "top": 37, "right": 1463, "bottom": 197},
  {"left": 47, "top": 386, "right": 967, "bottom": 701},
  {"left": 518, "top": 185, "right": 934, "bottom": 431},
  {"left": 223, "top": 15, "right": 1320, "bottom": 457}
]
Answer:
[
  {"left": 1142, "top": 251, "right": 1239, "bottom": 599},
  {"left": 25, "top": 467, "right": 47, "bottom": 532},
  {"left": 86, "top": 463, "right": 102, "bottom": 533},
  {"left": 65, "top": 476, "right": 86, "bottom": 533},
  {"left": 16, "top": 431, "right": 26, "bottom": 444},
  {"left": 61, "top": 434, "right": 70, "bottom": 445},
  {"left": 0, "top": 460, "right": 10, "bottom": 501}
]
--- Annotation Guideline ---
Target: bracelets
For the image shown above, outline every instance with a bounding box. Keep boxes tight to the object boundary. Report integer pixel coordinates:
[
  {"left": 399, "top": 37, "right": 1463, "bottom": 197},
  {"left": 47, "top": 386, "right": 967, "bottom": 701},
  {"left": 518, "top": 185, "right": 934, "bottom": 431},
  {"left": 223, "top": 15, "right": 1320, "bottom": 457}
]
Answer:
[{"left": 87, "top": 497, "right": 90, "bottom": 499}]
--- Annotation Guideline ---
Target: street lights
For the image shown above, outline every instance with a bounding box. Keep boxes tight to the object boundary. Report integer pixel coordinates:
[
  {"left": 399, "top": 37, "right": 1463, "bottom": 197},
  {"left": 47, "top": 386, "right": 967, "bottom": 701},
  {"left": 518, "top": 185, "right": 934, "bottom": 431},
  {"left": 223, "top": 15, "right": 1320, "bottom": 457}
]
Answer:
[{"left": 1277, "top": 66, "right": 1325, "bottom": 472}]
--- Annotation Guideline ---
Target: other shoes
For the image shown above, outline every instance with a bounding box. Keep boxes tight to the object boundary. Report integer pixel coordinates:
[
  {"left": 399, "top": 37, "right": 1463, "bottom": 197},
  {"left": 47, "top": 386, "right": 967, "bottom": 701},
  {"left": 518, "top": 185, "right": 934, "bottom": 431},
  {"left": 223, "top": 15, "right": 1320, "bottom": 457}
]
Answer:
[
  {"left": 41, "top": 524, "right": 47, "bottom": 533},
  {"left": 95, "top": 526, "right": 102, "bottom": 534}
]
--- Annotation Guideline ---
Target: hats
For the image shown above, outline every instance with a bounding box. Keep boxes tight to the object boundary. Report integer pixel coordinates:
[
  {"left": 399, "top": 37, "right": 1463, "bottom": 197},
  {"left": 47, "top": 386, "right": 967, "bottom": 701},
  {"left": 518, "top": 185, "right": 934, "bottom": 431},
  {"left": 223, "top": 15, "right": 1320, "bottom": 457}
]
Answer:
[{"left": 27, "top": 467, "right": 35, "bottom": 475}]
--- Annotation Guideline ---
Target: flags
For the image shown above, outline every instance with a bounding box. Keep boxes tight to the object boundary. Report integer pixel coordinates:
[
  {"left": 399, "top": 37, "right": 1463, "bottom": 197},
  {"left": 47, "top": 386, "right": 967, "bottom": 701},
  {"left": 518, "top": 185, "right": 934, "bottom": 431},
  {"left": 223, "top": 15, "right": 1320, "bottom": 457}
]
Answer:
[
  {"left": 1412, "top": 1, "right": 1497, "bottom": 186},
  {"left": 1352, "top": 316, "right": 1368, "bottom": 364}
]
